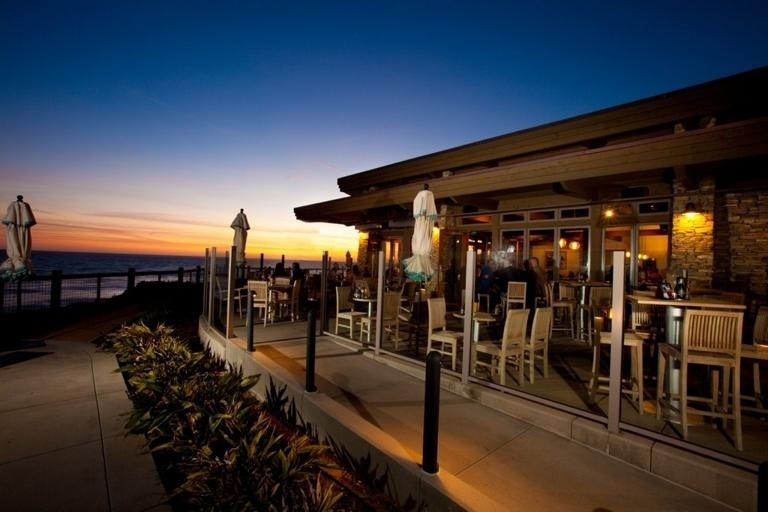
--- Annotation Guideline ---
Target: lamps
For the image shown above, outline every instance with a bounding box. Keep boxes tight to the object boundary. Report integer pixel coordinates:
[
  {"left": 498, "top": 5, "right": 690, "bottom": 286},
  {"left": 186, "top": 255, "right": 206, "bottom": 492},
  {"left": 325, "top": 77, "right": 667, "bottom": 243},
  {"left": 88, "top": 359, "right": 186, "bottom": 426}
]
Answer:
[{"left": 681, "top": 195, "right": 701, "bottom": 219}]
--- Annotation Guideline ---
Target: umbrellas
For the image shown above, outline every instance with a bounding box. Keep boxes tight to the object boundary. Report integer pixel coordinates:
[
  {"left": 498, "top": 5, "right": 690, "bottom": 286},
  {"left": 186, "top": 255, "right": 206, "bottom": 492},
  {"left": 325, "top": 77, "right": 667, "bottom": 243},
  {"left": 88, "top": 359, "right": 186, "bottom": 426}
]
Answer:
[
  {"left": 402, "top": 183, "right": 438, "bottom": 281},
  {"left": 0, "top": 195, "right": 37, "bottom": 283},
  {"left": 230, "top": 208, "right": 250, "bottom": 266}
]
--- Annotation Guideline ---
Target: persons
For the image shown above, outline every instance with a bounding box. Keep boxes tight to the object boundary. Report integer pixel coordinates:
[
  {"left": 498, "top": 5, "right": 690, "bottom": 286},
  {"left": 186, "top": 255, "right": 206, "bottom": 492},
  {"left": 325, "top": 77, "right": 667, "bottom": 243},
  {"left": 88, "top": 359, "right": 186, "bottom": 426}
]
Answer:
[
  {"left": 647, "top": 268, "right": 663, "bottom": 283},
  {"left": 528, "top": 257, "right": 546, "bottom": 297},
  {"left": 638, "top": 270, "right": 646, "bottom": 284},
  {"left": 272, "top": 261, "right": 363, "bottom": 318}
]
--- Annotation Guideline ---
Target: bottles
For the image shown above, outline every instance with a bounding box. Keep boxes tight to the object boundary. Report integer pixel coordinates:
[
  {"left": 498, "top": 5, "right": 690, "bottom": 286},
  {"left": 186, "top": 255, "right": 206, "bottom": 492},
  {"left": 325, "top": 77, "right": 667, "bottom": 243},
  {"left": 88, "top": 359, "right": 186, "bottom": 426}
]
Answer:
[{"left": 677, "top": 278, "right": 685, "bottom": 298}]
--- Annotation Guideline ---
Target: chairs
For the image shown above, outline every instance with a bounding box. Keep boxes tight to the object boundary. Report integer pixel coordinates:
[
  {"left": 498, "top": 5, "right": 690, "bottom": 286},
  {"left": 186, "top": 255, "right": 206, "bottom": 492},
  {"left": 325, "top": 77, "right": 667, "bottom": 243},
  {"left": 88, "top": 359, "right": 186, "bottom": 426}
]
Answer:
[
  {"left": 215, "top": 275, "right": 302, "bottom": 328},
  {"left": 334, "top": 273, "right": 767, "bottom": 451}
]
